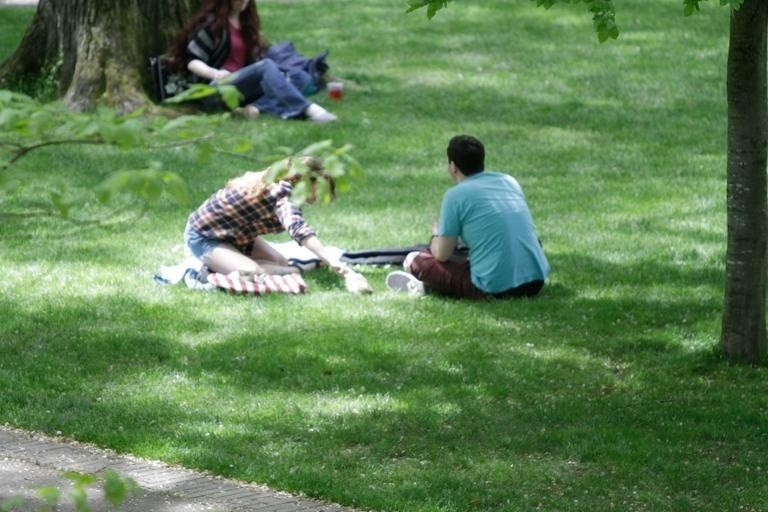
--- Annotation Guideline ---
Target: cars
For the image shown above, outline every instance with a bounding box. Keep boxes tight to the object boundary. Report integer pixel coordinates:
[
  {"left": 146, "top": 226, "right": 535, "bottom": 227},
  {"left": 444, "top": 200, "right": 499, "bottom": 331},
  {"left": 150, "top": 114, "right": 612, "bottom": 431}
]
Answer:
[{"left": 327, "top": 82, "right": 344, "bottom": 101}]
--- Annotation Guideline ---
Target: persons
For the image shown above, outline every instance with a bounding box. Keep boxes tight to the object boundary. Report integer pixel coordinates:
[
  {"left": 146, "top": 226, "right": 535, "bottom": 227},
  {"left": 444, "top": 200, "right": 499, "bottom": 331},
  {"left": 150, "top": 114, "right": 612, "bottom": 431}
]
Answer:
[
  {"left": 181, "top": 153, "right": 376, "bottom": 297},
  {"left": 170, "top": 0, "right": 339, "bottom": 123},
  {"left": 381, "top": 133, "right": 551, "bottom": 302}
]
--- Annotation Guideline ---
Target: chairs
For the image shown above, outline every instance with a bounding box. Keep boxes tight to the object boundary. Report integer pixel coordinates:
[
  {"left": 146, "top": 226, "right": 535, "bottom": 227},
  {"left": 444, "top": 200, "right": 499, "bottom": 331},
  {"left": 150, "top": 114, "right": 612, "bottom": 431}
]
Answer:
[
  {"left": 196, "top": 265, "right": 214, "bottom": 284},
  {"left": 385, "top": 270, "right": 428, "bottom": 297},
  {"left": 236, "top": 106, "right": 260, "bottom": 119},
  {"left": 309, "top": 111, "right": 337, "bottom": 124}
]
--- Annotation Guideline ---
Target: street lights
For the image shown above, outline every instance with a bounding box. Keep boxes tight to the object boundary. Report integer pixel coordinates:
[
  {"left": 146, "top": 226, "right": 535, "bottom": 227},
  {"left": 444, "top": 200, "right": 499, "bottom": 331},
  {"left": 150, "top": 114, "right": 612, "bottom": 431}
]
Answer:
[{"left": 266, "top": 40, "right": 329, "bottom": 98}]
